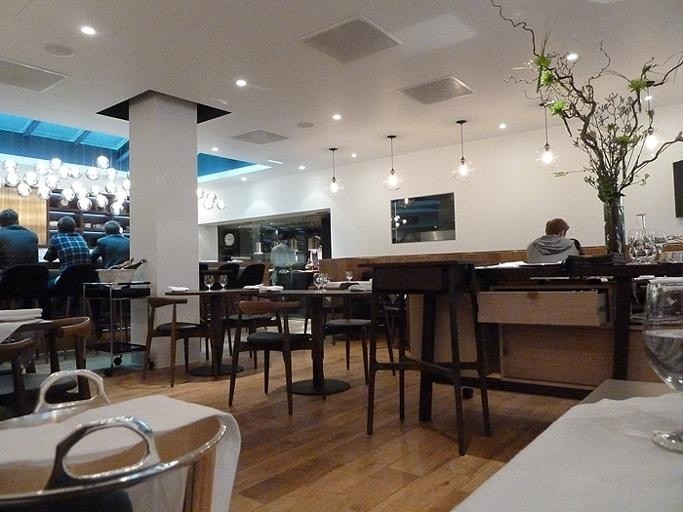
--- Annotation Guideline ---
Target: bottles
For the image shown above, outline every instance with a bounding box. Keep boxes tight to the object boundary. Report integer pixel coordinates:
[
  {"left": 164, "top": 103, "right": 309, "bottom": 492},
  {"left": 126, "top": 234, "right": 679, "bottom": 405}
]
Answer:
[
  {"left": 627, "top": 214, "right": 657, "bottom": 265},
  {"left": 105, "top": 258, "right": 146, "bottom": 269}
]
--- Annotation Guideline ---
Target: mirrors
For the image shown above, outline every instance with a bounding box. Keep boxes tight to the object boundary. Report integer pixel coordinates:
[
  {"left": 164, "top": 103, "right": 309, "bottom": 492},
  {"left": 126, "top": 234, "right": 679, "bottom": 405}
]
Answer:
[{"left": 391, "top": 193, "right": 455, "bottom": 244}]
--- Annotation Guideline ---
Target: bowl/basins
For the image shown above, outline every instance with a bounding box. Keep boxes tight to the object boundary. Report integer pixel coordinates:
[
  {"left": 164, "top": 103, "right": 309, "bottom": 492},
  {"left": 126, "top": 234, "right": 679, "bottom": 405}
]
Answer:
[{"left": 95, "top": 268, "right": 137, "bottom": 288}]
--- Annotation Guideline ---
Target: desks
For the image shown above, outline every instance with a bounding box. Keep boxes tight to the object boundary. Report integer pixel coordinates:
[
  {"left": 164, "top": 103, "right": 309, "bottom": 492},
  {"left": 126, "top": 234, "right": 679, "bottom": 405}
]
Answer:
[
  {"left": 360, "top": 261, "right": 491, "bottom": 456},
  {"left": 449, "top": 380, "right": 683, "bottom": 512}
]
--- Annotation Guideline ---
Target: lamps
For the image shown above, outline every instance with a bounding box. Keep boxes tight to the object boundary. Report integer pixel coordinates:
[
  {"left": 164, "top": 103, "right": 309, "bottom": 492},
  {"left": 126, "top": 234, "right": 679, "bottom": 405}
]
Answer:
[
  {"left": 384, "top": 135, "right": 402, "bottom": 190},
  {"left": 535, "top": 103, "right": 558, "bottom": 172},
  {"left": 324, "top": 147, "right": 344, "bottom": 198},
  {"left": 645, "top": 81, "right": 658, "bottom": 150},
  {"left": 453, "top": 121, "right": 473, "bottom": 180}
]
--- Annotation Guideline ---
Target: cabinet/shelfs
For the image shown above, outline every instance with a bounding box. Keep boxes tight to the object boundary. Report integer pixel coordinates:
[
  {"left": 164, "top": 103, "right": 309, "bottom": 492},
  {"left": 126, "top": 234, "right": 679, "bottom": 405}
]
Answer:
[{"left": 46, "top": 188, "right": 130, "bottom": 248}]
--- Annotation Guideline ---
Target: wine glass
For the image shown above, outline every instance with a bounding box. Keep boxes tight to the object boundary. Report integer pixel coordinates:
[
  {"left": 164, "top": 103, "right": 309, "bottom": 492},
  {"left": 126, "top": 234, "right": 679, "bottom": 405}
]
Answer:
[
  {"left": 345, "top": 271, "right": 353, "bottom": 282},
  {"left": 641, "top": 276, "right": 683, "bottom": 453},
  {"left": 204, "top": 275, "right": 214, "bottom": 291},
  {"left": 312, "top": 272, "right": 328, "bottom": 293},
  {"left": 218, "top": 275, "right": 228, "bottom": 291}
]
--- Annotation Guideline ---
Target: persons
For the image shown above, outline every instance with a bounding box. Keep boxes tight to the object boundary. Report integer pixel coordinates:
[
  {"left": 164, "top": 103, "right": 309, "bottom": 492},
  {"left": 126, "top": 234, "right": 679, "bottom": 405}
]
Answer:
[
  {"left": 89, "top": 220, "right": 130, "bottom": 283},
  {"left": 570, "top": 238, "right": 584, "bottom": 256},
  {"left": 270, "top": 233, "right": 296, "bottom": 290},
  {"left": 41, "top": 216, "right": 91, "bottom": 320},
  {"left": 0, "top": 208, "right": 39, "bottom": 281},
  {"left": 527, "top": 218, "right": 577, "bottom": 265}
]
